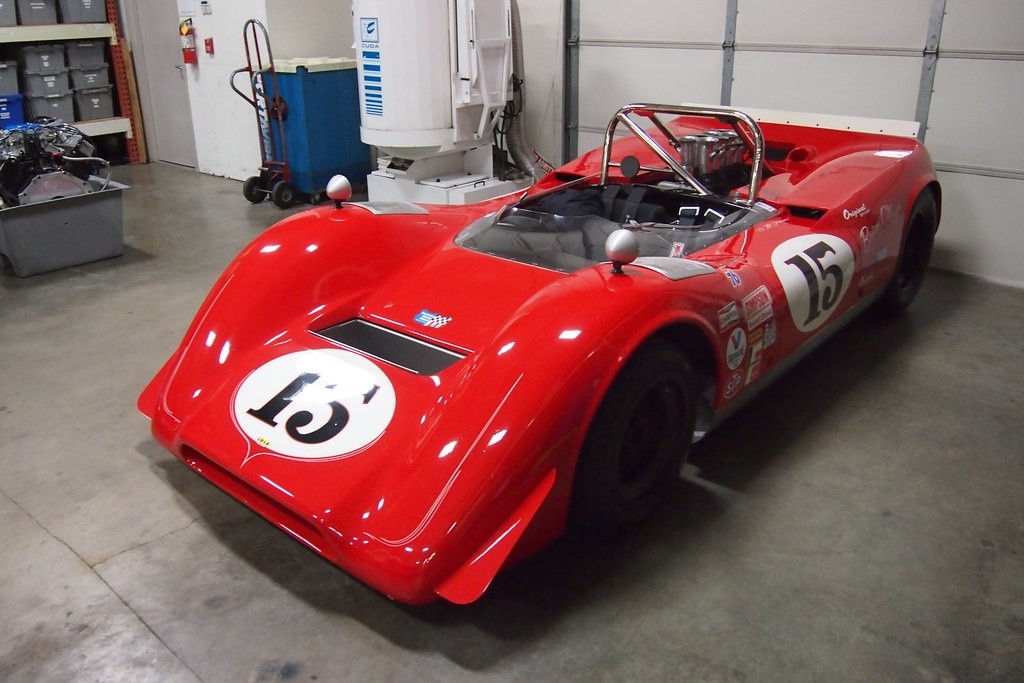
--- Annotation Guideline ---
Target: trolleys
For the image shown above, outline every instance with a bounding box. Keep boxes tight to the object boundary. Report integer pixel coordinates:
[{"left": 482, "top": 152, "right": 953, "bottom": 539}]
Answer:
[{"left": 229, "top": 19, "right": 299, "bottom": 208}]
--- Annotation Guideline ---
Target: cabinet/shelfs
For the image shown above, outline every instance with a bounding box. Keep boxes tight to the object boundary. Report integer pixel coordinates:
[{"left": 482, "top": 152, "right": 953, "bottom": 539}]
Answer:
[{"left": 0, "top": 0, "right": 142, "bottom": 166}]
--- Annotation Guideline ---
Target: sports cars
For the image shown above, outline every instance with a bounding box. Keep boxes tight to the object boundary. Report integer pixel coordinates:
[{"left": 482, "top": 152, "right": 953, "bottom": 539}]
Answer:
[{"left": 137, "top": 101, "right": 941, "bottom": 598}]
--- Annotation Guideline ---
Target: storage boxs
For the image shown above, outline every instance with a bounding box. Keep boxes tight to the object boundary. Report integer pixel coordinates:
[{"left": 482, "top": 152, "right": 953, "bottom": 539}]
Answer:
[{"left": 0, "top": 0, "right": 114, "bottom": 130}]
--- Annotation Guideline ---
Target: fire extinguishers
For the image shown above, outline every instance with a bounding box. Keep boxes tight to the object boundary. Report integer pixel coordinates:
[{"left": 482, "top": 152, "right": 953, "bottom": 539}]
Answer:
[{"left": 179, "top": 18, "right": 198, "bottom": 64}]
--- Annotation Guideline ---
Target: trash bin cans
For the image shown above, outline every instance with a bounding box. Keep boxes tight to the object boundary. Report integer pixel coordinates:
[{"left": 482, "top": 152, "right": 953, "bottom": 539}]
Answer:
[{"left": 252, "top": 57, "right": 373, "bottom": 204}]
